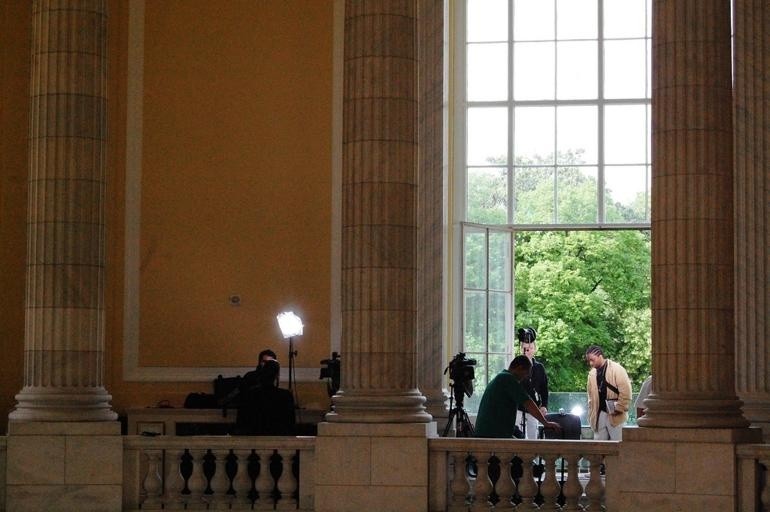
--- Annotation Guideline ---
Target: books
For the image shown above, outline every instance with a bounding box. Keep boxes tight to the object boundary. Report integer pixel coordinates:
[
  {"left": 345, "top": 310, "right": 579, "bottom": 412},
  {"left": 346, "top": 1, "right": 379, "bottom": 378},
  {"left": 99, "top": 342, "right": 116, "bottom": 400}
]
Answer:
[{"left": 604, "top": 398, "right": 618, "bottom": 416}]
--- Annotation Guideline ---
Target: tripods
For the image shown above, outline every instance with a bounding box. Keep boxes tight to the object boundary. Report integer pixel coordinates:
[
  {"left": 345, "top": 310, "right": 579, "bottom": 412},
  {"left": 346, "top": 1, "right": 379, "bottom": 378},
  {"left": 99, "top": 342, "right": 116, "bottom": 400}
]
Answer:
[{"left": 442, "top": 382, "right": 475, "bottom": 437}]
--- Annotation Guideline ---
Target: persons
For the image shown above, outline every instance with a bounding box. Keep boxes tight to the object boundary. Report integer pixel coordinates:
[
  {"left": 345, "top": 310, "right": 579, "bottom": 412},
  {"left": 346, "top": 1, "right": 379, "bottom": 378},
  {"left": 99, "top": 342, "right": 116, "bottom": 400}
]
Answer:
[
  {"left": 236, "top": 359, "right": 296, "bottom": 502},
  {"left": 515, "top": 341, "right": 549, "bottom": 466},
  {"left": 586, "top": 347, "right": 632, "bottom": 477},
  {"left": 476, "top": 356, "right": 561, "bottom": 504},
  {"left": 634, "top": 374, "right": 653, "bottom": 418},
  {"left": 236, "top": 350, "right": 277, "bottom": 408}
]
